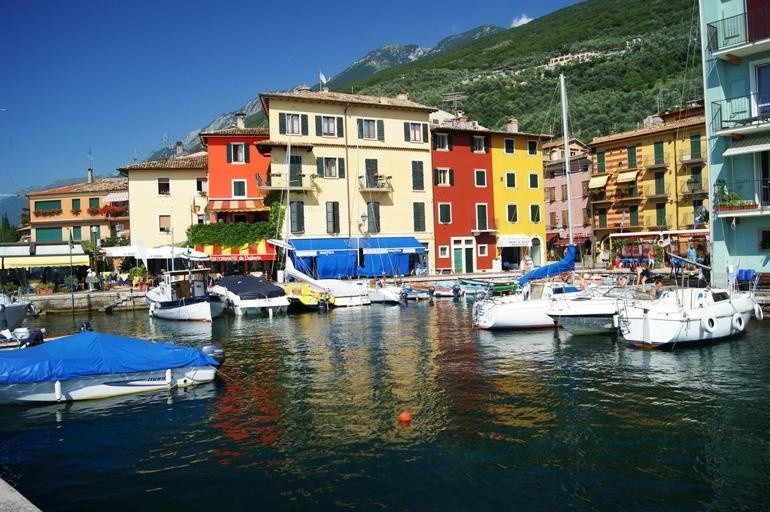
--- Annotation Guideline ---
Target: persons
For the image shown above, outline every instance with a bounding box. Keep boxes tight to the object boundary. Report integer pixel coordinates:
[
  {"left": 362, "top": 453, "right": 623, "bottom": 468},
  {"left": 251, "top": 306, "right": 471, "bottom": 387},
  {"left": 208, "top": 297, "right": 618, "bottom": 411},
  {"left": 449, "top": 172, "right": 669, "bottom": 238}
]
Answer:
[
  {"left": 631, "top": 266, "right": 649, "bottom": 293},
  {"left": 648, "top": 247, "right": 656, "bottom": 269},
  {"left": 687, "top": 243, "right": 697, "bottom": 270},
  {"left": 647, "top": 279, "right": 664, "bottom": 296},
  {"left": 616, "top": 276, "right": 627, "bottom": 288}
]
